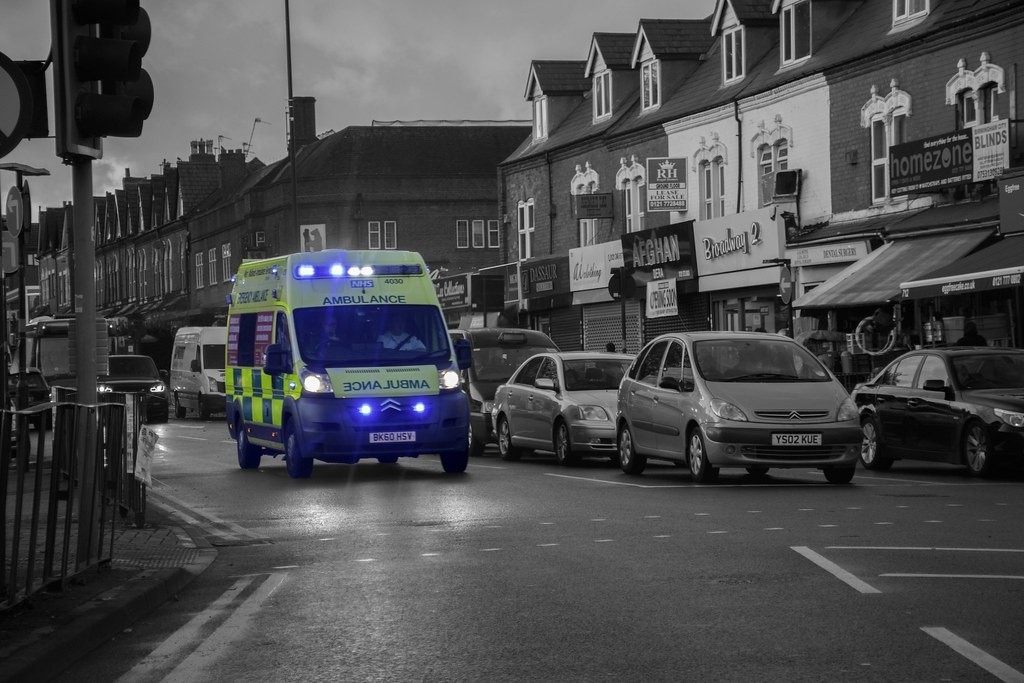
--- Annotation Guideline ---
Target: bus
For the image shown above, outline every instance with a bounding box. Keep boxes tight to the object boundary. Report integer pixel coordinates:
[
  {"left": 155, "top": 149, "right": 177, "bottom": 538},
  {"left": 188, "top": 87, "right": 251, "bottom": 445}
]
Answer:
[{"left": 8, "top": 316, "right": 76, "bottom": 430}]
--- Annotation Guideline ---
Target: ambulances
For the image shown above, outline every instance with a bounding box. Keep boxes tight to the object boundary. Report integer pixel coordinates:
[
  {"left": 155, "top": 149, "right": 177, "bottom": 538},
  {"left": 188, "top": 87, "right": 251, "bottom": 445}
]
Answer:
[{"left": 221, "top": 249, "right": 476, "bottom": 473}]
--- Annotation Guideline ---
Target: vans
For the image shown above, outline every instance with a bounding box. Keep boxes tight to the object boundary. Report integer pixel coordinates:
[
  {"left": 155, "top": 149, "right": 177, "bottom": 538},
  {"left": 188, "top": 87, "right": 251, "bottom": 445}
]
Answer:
[
  {"left": 171, "top": 326, "right": 230, "bottom": 418},
  {"left": 447, "top": 329, "right": 584, "bottom": 455}
]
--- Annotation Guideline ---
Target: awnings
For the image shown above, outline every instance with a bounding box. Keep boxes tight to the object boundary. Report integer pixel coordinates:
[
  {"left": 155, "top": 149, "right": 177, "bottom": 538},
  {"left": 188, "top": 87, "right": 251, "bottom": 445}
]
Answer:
[
  {"left": 790, "top": 227, "right": 997, "bottom": 308},
  {"left": 901, "top": 235, "right": 1023, "bottom": 302}
]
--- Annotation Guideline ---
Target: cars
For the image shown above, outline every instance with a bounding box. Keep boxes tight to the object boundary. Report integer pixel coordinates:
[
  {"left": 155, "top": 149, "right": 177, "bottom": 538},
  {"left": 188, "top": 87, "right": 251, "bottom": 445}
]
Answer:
[
  {"left": 491, "top": 350, "right": 638, "bottom": 464},
  {"left": 9, "top": 369, "right": 51, "bottom": 428},
  {"left": 94, "top": 355, "right": 170, "bottom": 425},
  {"left": 616, "top": 329, "right": 865, "bottom": 484},
  {"left": 852, "top": 346, "right": 1024, "bottom": 476}
]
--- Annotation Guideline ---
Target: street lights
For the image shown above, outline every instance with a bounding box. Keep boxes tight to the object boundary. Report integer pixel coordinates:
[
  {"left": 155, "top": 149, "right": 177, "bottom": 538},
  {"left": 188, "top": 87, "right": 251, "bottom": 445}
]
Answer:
[
  {"left": 1, "top": 162, "right": 51, "bottom": 466},
  {"left": 764, "top": 259, "right": 794, "bottom": 341},
  {"left": 610, "top": 269, "right": 635, "bottom": 353}
]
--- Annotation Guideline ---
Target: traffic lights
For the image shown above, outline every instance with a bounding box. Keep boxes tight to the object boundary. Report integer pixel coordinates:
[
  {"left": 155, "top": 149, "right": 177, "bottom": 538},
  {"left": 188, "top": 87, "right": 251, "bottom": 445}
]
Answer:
[{"left": 61, "top": 0, "right": 154, "bottom": 156}]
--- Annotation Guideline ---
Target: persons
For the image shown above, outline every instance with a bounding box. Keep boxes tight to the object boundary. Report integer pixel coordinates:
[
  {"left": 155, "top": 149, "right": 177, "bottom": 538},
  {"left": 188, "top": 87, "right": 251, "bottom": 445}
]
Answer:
[
  {"left": 596, "top": 343, "right": 623, "bottom": 377},
  {"left": 697, "top": 349, "right": 723, "bottom": 380},
  {"left": 303, "top": 315, "right": 348, "bottom": 355},
  {"left": 953, "top": 321, "right": 988, "bottom": 346},
  {"left": 378, "top": 313, "right": 425, "bottom": 352}
]
